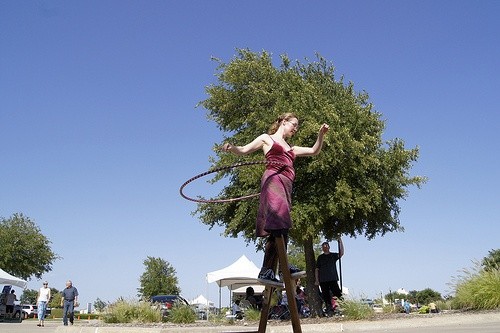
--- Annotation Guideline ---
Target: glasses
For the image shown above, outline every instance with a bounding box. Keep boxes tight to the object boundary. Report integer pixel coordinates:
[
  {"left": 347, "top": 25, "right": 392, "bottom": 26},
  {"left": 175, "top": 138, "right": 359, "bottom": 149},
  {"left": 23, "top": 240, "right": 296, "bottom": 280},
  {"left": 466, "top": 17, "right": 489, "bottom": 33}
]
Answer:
[{"left": 286, "top": 120, "right": 297, "bottom": 127}]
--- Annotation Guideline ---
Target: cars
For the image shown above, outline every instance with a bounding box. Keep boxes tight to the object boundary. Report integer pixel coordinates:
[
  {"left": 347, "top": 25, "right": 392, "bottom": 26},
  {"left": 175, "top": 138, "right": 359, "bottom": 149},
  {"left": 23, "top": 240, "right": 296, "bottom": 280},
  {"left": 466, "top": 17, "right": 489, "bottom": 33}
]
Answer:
[
  {"left": 150, "top": 294, "right": 189, "bottom": 316},
  {"left": 0, "top": 298, "right": 21, "bottom": 320}
]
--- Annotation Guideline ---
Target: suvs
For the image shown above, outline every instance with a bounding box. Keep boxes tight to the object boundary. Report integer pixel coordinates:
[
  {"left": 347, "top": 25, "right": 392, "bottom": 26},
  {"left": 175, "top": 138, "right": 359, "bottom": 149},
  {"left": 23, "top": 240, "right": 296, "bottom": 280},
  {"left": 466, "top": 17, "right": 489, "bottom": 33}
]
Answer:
[{"left": 20, "top": 303, "right": 38, "bottom": 319}]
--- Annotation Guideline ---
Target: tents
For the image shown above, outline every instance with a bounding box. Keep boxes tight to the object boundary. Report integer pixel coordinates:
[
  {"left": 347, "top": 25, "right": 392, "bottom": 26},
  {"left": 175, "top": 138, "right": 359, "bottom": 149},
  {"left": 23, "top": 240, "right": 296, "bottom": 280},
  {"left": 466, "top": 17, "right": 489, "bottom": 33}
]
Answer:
[
  {"left": 205, "top": 255, "right": 265, "bottom": 309},
  {"left": 191, "top": 295, "right": 214, "bottom": 307},
  {"left": 0, "top": 268, "right": 27, "bottom": 322}
]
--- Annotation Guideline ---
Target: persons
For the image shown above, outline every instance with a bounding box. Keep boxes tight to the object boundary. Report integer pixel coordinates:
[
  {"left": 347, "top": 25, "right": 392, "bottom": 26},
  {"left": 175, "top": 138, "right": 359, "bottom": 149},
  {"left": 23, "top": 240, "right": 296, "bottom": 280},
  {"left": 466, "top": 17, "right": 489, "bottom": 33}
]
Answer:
[
  {"left": 315, "top": 238, "right": 345, "bottom": 314},
  {"left": 404, "top": 299, "right": 410, "bottom": 314},
  {"left": 60, "top": 280, "right": 79, "bottom": 325},
  {"left": 222, "top": 113, "right": 329, "bottom": 286},
  {"left": 5, "top": 290, "right": 18, "bottom": 320},
  {"left": 232, "top": 300, "right": 241, "bottom": 315},
  {"left": 245, "top": 287, "right": 262, "bottom": 308},
  {"left": 36, "top": 281, "right": 51, "bottom": 327}
]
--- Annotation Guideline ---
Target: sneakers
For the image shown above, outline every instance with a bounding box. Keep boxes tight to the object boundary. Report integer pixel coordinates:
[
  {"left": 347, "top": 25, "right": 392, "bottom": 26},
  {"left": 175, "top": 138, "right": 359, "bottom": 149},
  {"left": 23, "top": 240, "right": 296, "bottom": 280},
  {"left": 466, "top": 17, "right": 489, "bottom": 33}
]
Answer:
[
  {"left": 257, "top": 266, "right": 283, "bottom": 286},
  {"left": 278, "top": 265, "right": 306, "bottom": 281}
]
